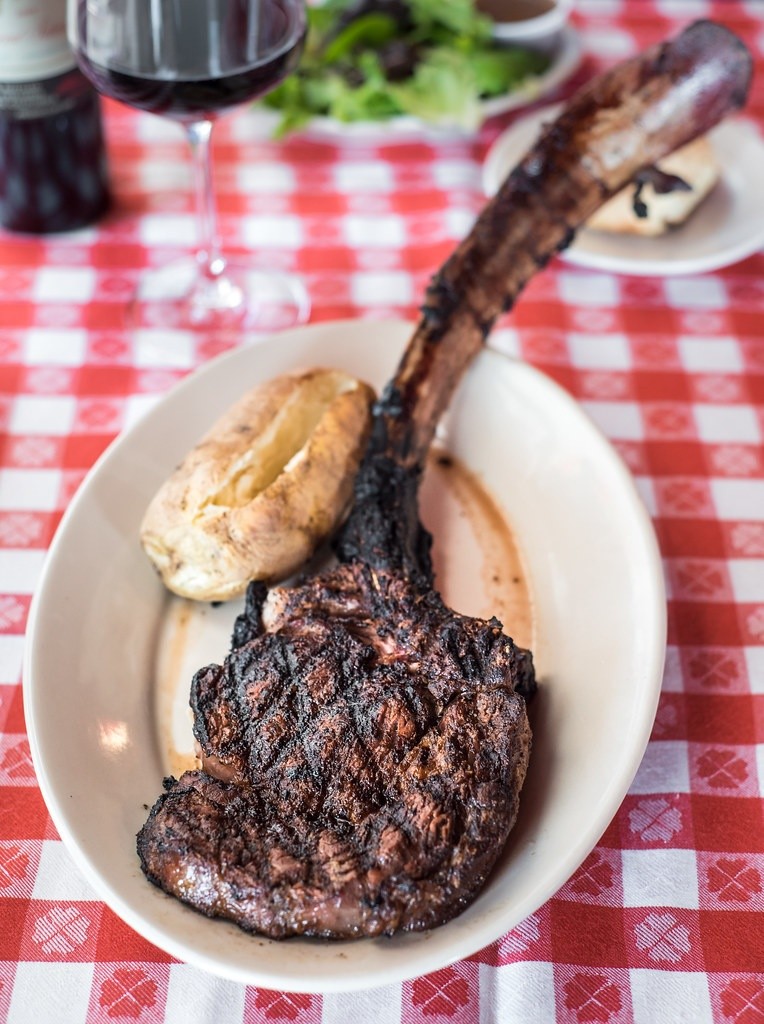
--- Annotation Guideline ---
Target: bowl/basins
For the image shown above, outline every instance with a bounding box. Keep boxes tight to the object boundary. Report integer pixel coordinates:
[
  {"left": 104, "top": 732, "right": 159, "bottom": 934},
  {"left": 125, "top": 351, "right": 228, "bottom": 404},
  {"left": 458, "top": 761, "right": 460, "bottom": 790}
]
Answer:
[{"left": 489, "top": 0, "right": 573, "bottom": 39}]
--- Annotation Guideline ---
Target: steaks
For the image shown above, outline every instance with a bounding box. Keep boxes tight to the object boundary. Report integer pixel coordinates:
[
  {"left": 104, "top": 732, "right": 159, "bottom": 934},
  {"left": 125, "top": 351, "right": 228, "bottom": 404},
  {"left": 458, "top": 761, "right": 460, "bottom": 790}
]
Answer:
[{"left": 130, "top": 18, "right": 759, "bottom": 939}]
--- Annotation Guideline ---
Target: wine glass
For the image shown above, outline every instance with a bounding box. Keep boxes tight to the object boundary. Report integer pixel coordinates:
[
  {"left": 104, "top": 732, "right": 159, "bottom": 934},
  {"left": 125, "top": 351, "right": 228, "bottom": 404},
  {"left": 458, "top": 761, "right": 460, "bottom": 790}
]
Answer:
[{"left": 64, "top": 1, "right": 311, "bottom": 342}]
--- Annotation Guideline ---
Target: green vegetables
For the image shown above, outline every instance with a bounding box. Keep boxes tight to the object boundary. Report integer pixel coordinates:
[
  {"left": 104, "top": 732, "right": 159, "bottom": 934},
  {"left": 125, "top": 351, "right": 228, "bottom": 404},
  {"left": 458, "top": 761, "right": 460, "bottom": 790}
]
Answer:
[{"left": 260, "top": 0, "right": 546, "bottom": 142}]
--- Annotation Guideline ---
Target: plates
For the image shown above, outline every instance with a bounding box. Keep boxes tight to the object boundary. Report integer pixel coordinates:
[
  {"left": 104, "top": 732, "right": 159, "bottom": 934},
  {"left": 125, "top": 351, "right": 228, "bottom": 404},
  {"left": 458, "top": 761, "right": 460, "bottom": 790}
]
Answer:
[
  {"left": 481, "top": 99, "right": 764, "bottom": 278},
  {"left": 21, "top": 316, "right": 668, "bottom": 995},
  {"left": 254, "top": 27, "right": 583, "bottom": 146}
]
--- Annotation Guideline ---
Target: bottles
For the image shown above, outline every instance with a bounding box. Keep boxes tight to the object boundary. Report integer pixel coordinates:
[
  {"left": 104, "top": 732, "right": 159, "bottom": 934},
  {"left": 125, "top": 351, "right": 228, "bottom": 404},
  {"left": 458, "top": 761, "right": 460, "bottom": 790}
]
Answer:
[{"left": 0, "top": 0, "right": 110, "bottom": 235}]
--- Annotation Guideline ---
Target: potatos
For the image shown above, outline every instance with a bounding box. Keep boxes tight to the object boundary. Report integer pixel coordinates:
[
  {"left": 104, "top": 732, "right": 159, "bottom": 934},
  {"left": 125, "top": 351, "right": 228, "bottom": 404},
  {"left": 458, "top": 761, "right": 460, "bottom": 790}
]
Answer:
[{"left": 141, "top": 364, "right": 378, "bottom": 602}]
